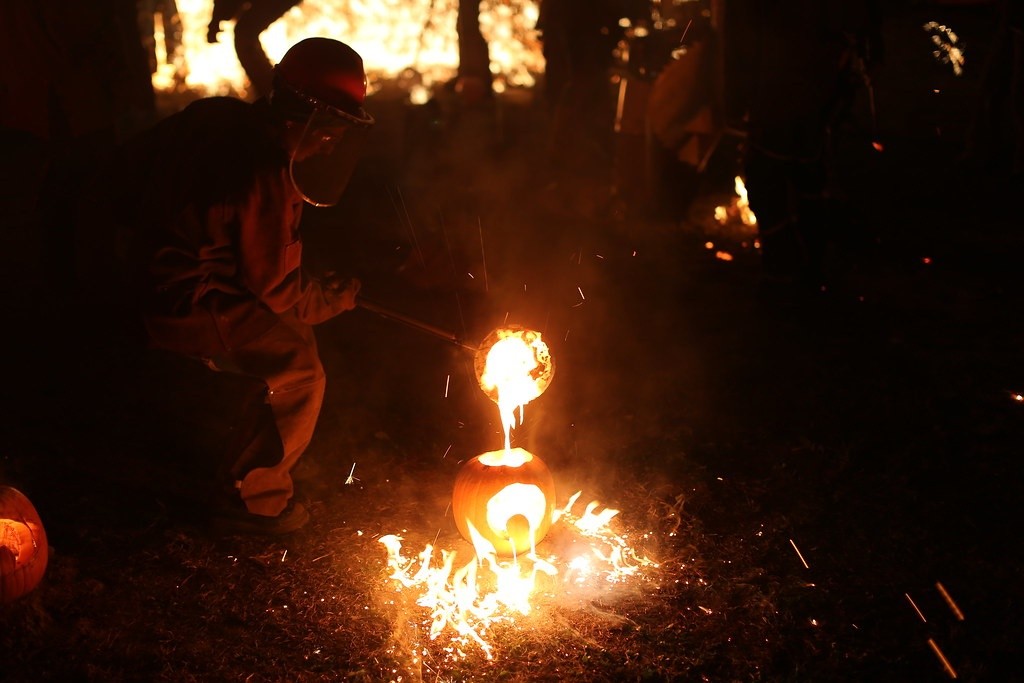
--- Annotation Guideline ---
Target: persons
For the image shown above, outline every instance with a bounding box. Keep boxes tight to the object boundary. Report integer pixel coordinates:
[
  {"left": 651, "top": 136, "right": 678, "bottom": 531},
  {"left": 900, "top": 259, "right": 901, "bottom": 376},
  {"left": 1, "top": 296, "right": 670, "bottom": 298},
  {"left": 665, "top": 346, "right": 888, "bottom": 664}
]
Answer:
[{"left": 77, "top": 38, "right": 376, "bottom": 535}]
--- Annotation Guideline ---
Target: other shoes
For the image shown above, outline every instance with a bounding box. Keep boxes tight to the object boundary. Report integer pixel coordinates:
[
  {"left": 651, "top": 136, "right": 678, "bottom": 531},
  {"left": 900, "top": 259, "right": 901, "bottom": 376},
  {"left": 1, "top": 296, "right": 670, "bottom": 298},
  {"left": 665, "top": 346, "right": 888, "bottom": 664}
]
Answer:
[{"left": 212, "top": 500, "right": 312, "bottom": 537}]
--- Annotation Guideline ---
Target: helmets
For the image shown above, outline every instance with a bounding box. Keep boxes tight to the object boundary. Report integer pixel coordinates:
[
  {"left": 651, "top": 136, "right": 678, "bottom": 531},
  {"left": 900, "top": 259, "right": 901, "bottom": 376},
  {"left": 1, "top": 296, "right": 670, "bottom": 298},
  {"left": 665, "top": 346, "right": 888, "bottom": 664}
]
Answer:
[{"left": 271, "top": 38, "right": 377, "bottom": 207}]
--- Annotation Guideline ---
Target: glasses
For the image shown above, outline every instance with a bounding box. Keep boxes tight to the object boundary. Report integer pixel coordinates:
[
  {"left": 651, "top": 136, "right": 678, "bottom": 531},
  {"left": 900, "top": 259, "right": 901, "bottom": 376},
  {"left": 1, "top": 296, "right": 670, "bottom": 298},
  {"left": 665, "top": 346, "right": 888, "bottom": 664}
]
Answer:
[{"left": 307, "top": 127, "right": 346, "bottom": 148}]
